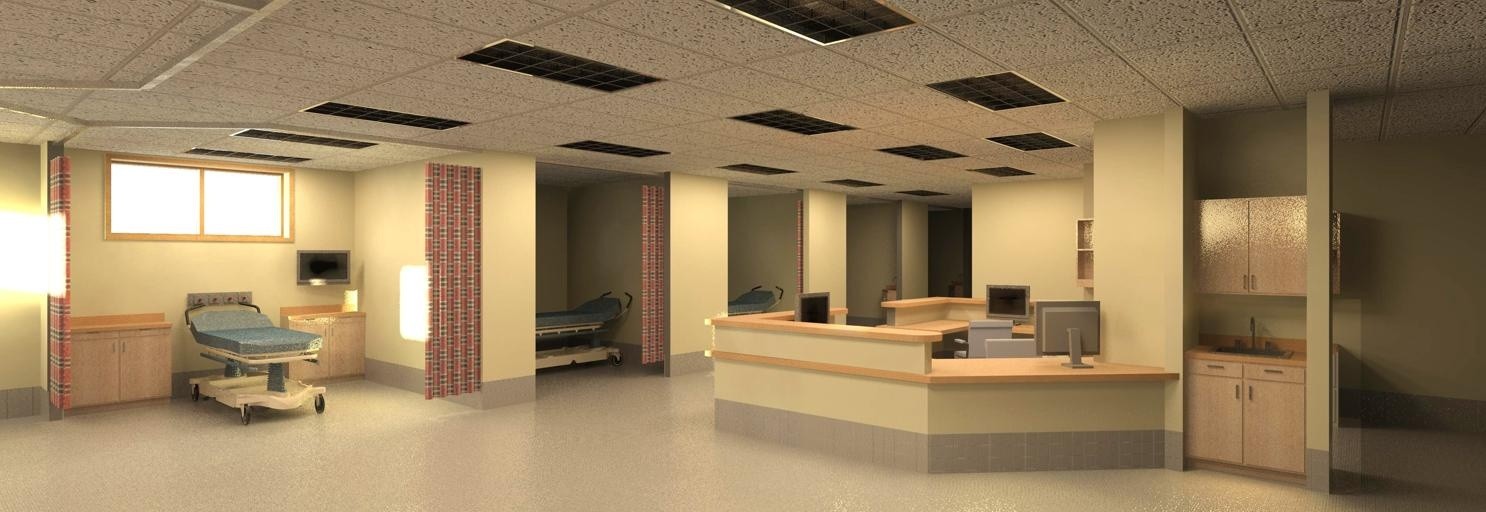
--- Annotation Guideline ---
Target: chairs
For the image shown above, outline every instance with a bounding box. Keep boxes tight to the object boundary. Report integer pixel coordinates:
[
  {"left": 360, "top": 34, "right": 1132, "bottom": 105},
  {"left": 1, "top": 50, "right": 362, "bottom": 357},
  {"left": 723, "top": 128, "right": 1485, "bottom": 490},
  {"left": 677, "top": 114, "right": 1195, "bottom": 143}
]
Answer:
[{"left": 952, "top": 321, "right": 1036, "bottom": 358}]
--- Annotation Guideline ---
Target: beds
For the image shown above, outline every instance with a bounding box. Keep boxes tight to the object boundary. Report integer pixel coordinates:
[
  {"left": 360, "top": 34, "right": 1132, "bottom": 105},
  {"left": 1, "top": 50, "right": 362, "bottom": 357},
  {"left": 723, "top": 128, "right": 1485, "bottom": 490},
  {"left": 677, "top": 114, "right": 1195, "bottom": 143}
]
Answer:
[
  {"left": 537, "top": 292, "right": 632, "bottom": 372},
  {"left": 185, "top": 303, "right": 324, "bottom": 425},
  {"left": 729, "top": 283, "right": 784, "bottom": 315}
]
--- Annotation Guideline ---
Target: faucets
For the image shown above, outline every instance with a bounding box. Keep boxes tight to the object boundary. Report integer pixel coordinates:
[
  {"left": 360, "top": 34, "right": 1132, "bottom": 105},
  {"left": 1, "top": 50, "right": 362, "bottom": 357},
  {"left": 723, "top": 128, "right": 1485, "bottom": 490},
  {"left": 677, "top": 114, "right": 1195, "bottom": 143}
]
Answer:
[{"left": 1250, "top": 316, "right": 1257, "bottom": 350}]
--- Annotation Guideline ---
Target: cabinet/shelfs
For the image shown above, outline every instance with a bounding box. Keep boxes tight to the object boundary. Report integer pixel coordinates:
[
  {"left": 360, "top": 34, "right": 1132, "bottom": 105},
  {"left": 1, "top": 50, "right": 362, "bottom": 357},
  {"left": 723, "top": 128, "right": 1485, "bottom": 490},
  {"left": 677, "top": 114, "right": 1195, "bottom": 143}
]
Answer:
[
  {"left": 281, "top": 305, "right": 366, "bottom": 387},
  {"left": 1192, "top": 195, "right": 1307, "bottom": 296},
  {"left": 63, "top": 312, "right": 173, "bottom": 415},
  {"left": 1186, "top": 359, "right": 1304, "bottom": 487},
  {"left": 1074, "top": 219, "right": 1095, "bottom": 289}
]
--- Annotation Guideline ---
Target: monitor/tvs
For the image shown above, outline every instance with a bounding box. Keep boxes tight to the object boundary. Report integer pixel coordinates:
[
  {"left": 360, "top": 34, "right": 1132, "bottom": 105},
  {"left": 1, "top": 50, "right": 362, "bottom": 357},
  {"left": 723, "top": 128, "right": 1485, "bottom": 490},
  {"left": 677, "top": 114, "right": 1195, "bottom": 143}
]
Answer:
[
  {"left": 1034, "top": 301, "right": 1100, "bottom": 369},
  {"left": 794, "top": 292, "right": 830, "bottom": 323},
  {"left": 986, "top": 285, "right": 1030, "bottom": 326},
  {"left": 297, "top": 250, "right": 350, "bottom": 285}
]
